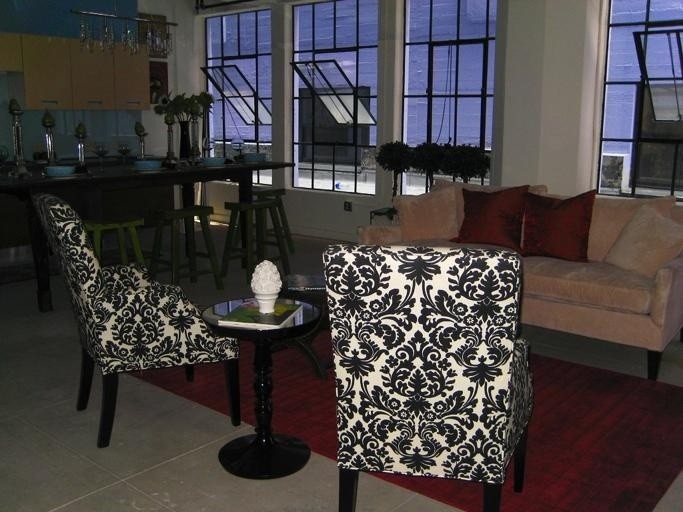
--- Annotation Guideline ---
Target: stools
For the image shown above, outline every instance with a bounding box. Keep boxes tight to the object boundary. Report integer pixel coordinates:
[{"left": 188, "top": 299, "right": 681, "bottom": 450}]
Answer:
[{"left": 81, "top": 186, "right": 295, "bottom": 290}]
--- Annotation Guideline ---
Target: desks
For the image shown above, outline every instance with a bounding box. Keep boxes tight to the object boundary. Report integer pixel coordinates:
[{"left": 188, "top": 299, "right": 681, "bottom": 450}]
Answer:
[{"left": 0, "top": 158, "right": 294, "bottom": 313}]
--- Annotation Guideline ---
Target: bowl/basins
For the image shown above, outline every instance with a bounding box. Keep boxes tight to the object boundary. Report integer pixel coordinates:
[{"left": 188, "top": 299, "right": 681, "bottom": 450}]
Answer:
[
  {"left": 43, "top": 165, "right": 75, "bottom": 176},
  {"left": 134, "top": 160, "right": 162, "bottom": 169},
  {"left": 200, "top": 156, "right": 225, "bottom": 165},
  {"left": 243, "top": 152, "right": 266, "bottom": 161}
]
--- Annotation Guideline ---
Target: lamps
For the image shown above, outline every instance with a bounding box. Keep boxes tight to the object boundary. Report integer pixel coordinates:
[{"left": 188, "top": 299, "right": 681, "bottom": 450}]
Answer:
[{"left": 70, "top": 0, "right": 179, "bottom": 57}]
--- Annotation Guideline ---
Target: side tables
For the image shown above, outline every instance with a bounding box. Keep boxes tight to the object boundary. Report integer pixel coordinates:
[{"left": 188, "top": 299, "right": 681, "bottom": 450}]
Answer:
[{"left": 247, "top": 254, "right": 334, "bottom": 379}]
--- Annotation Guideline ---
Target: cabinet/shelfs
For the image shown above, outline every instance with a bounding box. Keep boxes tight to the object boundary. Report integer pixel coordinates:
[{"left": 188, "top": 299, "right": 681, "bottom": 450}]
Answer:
[{"left": 0, "top": 0, "right": 150, "bottom": 110}]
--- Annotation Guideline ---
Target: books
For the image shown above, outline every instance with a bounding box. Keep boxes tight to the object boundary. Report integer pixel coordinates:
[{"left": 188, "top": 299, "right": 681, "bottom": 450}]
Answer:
[{"left": 218, "top": 301, "right": 303, "bottom": 329}]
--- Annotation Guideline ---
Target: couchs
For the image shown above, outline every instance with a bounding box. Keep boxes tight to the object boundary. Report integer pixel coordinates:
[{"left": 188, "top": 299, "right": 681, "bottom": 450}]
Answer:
[
  {"left": 357, "top": 179, "right": 683, "bottom": 381},
  {"left": 320, "top": 244, "right": 535, "bottom": 512}
]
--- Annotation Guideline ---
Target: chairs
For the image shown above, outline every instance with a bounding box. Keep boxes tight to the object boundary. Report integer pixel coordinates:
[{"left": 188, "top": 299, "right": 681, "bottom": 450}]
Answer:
[{"left": 32, "top": 192, "right": 241, "bottom": 448}]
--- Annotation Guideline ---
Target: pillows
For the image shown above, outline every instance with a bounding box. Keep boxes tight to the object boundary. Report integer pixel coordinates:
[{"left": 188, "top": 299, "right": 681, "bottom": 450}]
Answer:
[{"left": 391, "top": 178, "right": 683, "bottom": 280}]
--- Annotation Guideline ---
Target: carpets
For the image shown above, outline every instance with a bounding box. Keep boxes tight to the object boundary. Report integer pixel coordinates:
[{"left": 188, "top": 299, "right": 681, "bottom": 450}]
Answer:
[{"left": 127, "top": 330, "right": 683, "bottom": 512}]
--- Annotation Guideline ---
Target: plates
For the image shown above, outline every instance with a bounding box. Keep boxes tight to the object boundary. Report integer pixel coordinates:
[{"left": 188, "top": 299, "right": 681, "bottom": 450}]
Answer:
[
  {"left": 199, "top": 163, "right": 226, "bottom": 169},
  {"left": 130, "top": 167, "right": 170, "bottom": 175},
  {"left": 239, "top": 159, "right": 264, "bottom": 165},
  {"left": 41, "top": 173, "right": 80, "bottom": 181}
]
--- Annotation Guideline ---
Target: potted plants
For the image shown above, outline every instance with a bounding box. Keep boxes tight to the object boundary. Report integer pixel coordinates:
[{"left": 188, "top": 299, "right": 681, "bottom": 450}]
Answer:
[{"left": 7, "top": 90, "right": 214, "bottom": 178}]
